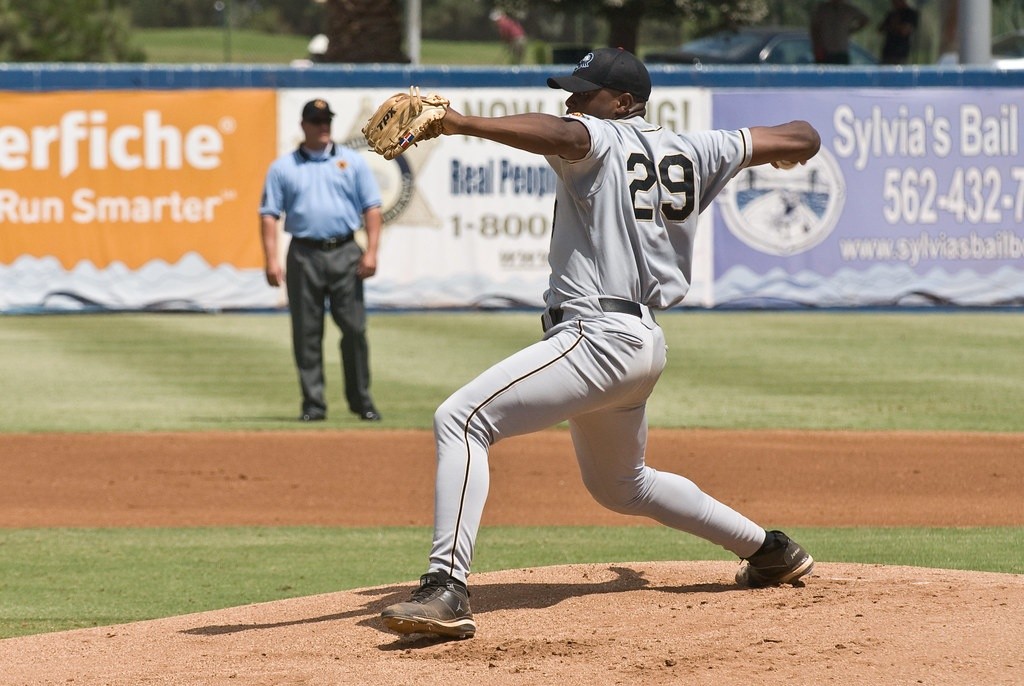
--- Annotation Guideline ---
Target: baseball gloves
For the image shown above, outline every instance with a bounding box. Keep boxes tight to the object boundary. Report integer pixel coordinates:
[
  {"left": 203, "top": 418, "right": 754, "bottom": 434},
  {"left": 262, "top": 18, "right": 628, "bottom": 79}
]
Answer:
[{"left": 360, "top": 84, "right": 451, "bottom": 160}]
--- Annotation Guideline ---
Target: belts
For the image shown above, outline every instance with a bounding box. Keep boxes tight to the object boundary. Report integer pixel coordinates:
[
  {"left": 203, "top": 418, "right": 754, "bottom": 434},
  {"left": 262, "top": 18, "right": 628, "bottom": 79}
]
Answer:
[
  {"left": 295, "top": 232, "right": 353, "bottom": 248},
  {"left": 541, "top": 298, "right": 655, "bottom": 333}
]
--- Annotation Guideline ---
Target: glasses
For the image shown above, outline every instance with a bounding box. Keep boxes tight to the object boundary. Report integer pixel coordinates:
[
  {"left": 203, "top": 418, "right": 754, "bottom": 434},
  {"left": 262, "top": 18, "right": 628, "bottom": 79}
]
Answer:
[{"left": 311, "top": 117, "right": 331, "bottom": 124}]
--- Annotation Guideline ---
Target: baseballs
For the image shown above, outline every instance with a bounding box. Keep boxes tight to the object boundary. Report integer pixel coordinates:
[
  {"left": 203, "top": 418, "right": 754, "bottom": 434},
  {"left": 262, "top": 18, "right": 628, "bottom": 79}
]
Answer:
[{"left": 776, "top": 159, "right": 798, "bottom": 170}]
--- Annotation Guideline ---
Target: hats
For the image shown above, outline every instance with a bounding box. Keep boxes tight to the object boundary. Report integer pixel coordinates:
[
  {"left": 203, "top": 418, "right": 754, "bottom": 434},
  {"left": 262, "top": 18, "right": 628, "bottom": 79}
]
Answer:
[
  {"left": 547, "top": 46, "right": 651, "bottom": 101},
  {"left": 302, "top": 99, "right": 335, "bottom": 119},
  {"left": 488, "top": 10, "right": 503, "bottom": 20}
]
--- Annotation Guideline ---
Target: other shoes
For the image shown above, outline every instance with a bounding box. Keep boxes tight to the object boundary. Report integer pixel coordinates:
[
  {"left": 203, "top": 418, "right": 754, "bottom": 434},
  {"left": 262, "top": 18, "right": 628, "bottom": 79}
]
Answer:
[
  {"left": 301, "top": 410, "right": 325, "bottom": 420},
  {"left": 349, "top": 403, "right": 381, "bottom": 420}
]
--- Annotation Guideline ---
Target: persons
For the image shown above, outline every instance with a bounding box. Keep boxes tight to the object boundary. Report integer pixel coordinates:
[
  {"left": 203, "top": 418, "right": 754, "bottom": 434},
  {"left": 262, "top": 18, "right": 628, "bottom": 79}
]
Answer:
[
  {"left": 260, "top": 97, "right": 380, "bottom": 421},
  {"left": 807, "top": 0, "right": 868, "bottom": 65},
  {"left": 489, "top": 10, "right": 527, "bottom": 65},
  {"left": 875, "top": 0, "right": 919, "bottom": 64},
  {"left": 600, "top": 0, "right": 642, "bottom": 56},
  {"left": 361, "top": 46, "right": 820, "bottom": 637}
]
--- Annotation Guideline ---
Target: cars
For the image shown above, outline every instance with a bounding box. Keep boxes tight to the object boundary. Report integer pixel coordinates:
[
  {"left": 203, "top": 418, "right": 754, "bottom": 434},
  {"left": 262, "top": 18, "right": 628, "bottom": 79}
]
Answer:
[
  {"left": 939, "top": 28, "right": 1024, "bottom": 66},
  {"left": 645, "top": 24, "right": 878, "bottom": 65}
]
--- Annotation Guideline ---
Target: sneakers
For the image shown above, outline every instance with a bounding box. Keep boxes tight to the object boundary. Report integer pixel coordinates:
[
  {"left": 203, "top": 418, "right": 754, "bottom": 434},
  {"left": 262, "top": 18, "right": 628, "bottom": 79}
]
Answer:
[
  {"left": 735, "top": 529, "right": 815, "bottom": 588},
  {"left": 381, "top": 568, "right": 477, "bottom": 638}
]
